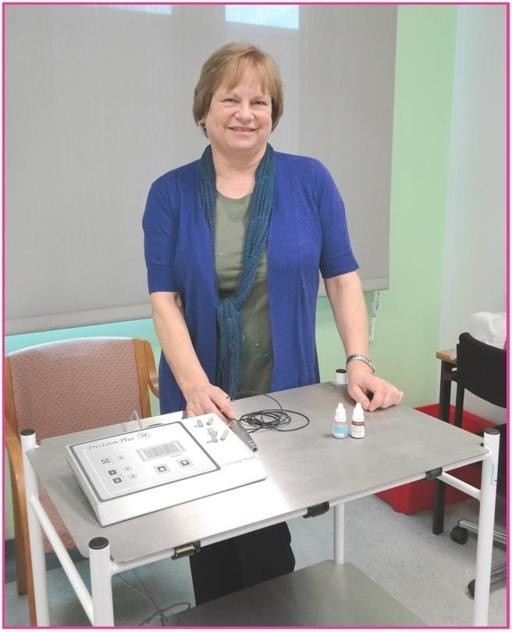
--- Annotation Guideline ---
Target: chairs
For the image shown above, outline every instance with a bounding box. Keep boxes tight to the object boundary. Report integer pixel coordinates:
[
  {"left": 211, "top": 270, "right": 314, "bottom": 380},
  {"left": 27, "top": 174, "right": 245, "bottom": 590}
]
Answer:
[
  {"left": 5, "top": 336, "right": 162, "bottom": 628},
  {"left": 450, "top": 331, "right": 505, "bottom": 598}
]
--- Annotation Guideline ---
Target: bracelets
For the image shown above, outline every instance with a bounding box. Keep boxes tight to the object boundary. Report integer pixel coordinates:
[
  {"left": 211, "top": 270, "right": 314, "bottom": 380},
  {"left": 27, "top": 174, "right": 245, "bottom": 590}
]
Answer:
[{"left": 346, "top": 353, "right": 375, "bottom": 373}]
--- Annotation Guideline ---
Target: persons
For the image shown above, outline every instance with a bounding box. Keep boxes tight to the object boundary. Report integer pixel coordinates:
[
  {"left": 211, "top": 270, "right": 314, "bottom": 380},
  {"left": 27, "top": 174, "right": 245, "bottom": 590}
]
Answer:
[{"left": 142, "top": 43, "right": 407, "bottom": 607}]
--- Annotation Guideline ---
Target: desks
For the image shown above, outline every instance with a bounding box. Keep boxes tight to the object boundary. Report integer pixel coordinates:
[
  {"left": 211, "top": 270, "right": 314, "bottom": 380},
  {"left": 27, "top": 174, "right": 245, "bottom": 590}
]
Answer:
[
  {"left": 22, "top": 369, "right": 501, "bottom": 626},
  {"left": 432, "top": 350, "right": 464, "bottom": 534}
]
